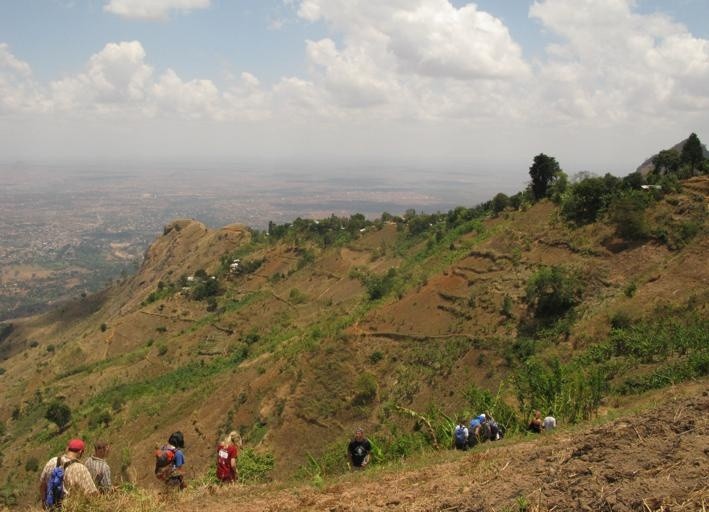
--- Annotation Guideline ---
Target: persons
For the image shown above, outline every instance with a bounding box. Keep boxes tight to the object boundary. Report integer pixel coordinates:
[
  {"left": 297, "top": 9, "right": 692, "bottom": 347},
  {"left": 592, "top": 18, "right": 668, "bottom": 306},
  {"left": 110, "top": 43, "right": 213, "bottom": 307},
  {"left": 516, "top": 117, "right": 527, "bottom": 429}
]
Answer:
[
  {"left": 451, "top": 409, "right": 506, "bottom": 450},
  {"left": 86, "top": 441, "right": 113, "bottom": 489},
  {"left": 152, "top": 430, "right": 186, "bottom": 488},
  {"left": 526, "top": 410, "right": 543, "bottom": 433},
  {"left": 35, "top": 439, "right": 100, "bottom": 510},
  {"left": 214, "top": 430, "right": 243, "bottom": 485},
  {"left": 346, "top": 427, "right": 373, "bottom": 469},
  {"left": 542, "top": 408, "right": 557, "bottom": 431}
]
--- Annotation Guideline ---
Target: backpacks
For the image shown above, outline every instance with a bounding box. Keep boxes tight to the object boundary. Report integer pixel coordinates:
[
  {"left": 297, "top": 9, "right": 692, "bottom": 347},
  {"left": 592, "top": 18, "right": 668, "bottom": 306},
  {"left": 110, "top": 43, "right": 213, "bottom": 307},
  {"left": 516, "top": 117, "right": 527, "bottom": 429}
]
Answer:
[
  {"left": 154, "top": 445, "right": 180, "bottom": 482},
  {"left": 44, "top": 456, "right": 80, "bottom": 511}
]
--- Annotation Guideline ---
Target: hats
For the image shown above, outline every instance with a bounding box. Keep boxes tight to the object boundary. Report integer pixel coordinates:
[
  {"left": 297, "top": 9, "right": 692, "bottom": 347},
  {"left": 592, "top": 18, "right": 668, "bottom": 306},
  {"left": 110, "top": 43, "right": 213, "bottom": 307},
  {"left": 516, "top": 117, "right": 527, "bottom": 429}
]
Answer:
[{"left": 69, "top": 439, "right": 84, "bottom": 452}]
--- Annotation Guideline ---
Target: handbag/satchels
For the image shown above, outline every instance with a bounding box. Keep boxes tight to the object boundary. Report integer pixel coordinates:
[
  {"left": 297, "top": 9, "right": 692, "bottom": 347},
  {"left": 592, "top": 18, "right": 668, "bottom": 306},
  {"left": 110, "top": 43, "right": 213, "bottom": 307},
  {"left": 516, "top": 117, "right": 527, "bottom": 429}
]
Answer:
[{"left": 455, "top": 426, "right": 466, "bottom": 444}]
